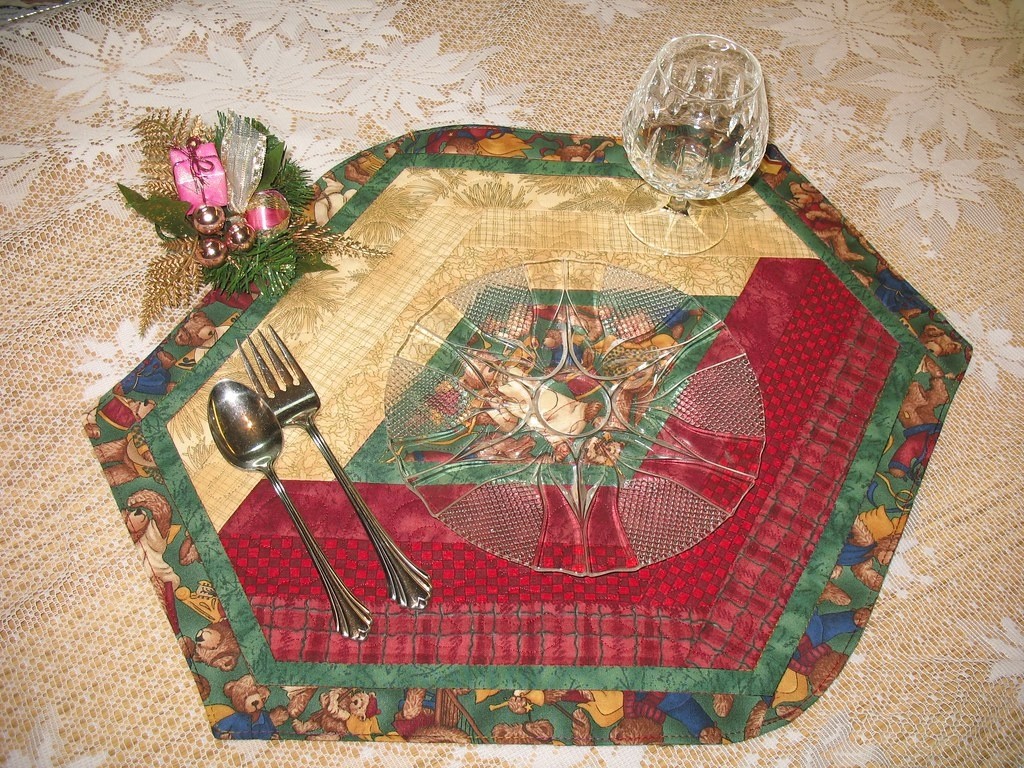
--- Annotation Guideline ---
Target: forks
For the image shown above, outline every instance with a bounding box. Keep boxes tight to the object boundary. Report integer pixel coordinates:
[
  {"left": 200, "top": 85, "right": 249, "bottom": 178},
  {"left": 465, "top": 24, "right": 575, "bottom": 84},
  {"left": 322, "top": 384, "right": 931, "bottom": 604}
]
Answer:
[{"left": 234, "top": 324, "right": 434, "bottom": 611}]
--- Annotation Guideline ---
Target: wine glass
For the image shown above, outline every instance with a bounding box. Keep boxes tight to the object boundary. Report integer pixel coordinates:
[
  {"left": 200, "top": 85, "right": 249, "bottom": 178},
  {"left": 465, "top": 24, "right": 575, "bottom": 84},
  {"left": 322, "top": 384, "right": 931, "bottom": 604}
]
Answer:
[{"left": 621, "top": 33, "right": 770, "bottom": 255}]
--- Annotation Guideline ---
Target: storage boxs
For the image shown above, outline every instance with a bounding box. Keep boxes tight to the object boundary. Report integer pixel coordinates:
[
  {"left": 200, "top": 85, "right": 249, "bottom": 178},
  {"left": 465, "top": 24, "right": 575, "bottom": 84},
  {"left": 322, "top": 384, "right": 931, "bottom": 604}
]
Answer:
[{"left": 170, "top": 136, "right": 231, "bottom": 217}]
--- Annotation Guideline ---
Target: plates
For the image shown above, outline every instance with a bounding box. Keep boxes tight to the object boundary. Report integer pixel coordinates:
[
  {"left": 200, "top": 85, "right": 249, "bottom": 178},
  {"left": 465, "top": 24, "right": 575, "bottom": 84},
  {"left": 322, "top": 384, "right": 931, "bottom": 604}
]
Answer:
[{"left": 383, "top": 259, "right": 767, "bottom": 578}]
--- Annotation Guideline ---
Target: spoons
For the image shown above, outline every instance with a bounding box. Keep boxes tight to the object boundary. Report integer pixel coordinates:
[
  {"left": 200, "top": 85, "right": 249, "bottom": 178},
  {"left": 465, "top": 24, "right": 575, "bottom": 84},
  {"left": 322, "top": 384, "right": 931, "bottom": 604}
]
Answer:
[{"left": 206, "top": 380, "right": 375, "bottom": 642}]
